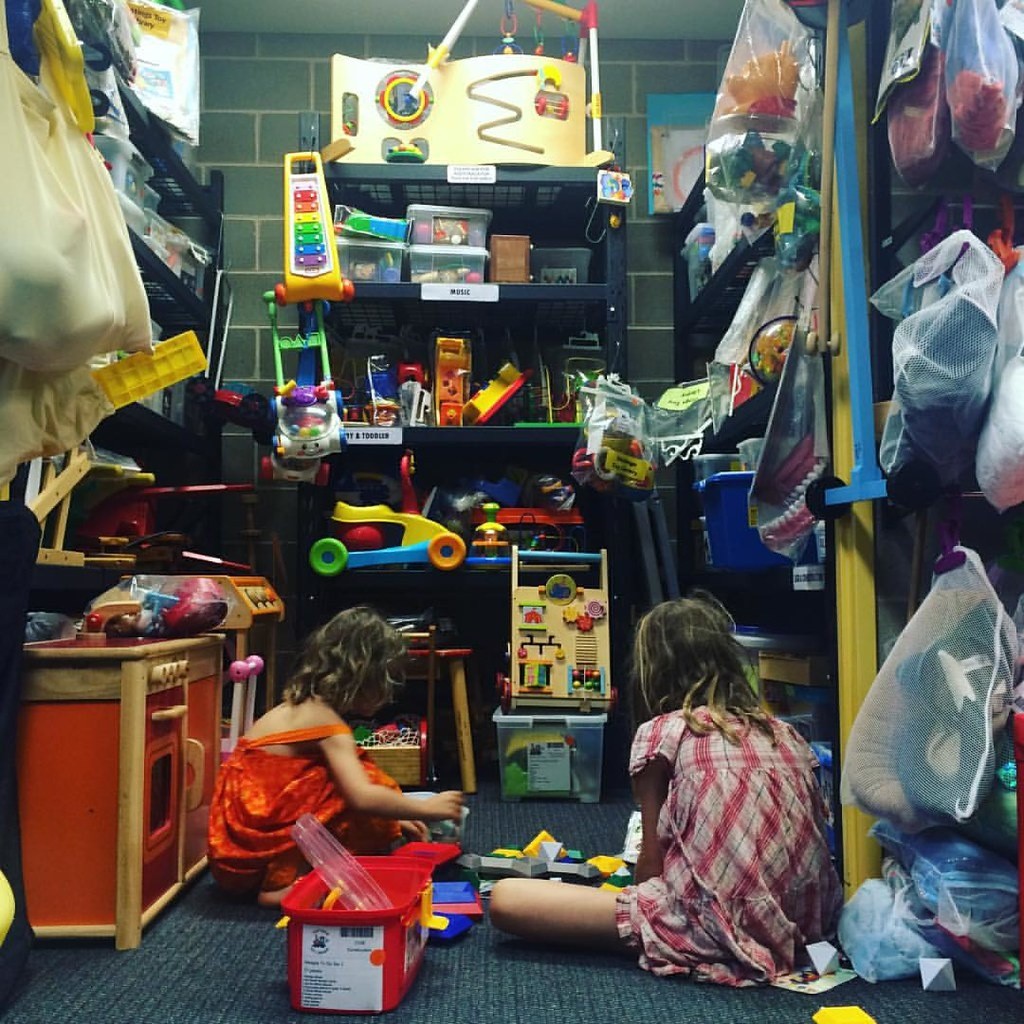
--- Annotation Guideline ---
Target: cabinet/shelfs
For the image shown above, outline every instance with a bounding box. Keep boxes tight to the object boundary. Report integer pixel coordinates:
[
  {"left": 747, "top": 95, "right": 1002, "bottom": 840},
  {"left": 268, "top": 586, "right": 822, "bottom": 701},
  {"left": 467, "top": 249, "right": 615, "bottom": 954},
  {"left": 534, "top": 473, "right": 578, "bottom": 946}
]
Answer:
[
  {"left": 293, "top": 111, "right": 635, "bottom": 801},
  {"left": 7, "top": 65, "right": 226, "bottom": 594},
  {"left": 16, "top": 633, "right": 226, "bottom": 951},
  {"left": 666, "top": 163, "right": 837, "bottom": 648}
]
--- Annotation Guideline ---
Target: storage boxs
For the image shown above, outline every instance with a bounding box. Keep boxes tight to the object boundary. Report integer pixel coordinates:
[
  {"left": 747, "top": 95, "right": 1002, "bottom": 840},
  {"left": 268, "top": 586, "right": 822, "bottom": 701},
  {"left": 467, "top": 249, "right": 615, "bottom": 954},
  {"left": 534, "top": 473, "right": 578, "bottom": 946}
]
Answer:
[
  {"left": 273, "top": 855, "right": 450, "bottom": 1014},
  {"left": 758, "top": 650, "right": 838, "bottom": 688},
  {"left": 690, "top": 438, "right": 826, "bottom": 570},
  {"left": 334, "top": 202, "right": 593, "bottom": 286},
  {"left": 491, "top": 704, "right": 608, "bottom": 803}
]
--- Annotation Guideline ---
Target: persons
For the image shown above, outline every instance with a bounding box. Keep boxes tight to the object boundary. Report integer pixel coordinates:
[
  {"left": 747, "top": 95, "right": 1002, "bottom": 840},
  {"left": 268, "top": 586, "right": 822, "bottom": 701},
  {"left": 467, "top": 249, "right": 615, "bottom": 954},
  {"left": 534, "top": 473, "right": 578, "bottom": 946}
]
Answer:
[
  {"left": 490, "top": 598, "right": 843, "bottom": 989},
  {"left": 200, "top": 601, "right": 464, "bottom": 909}
]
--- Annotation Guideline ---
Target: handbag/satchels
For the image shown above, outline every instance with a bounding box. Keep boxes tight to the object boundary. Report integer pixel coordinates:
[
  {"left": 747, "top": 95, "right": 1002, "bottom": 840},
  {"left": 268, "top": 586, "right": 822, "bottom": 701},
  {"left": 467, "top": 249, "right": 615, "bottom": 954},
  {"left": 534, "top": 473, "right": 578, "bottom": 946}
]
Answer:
[
  {"left": 571, "top": 255, "right": 830, "bottom": 563},
  {"left": 870, "top": 231, "right": 1024, "bottom": 513},
  {"left": 839, "top": 545, "right": 1018, "bottom": 835},
  {"left": 3, "top": 3, "right": 154, "bottom": 368},
  {"left": 888, "top": 0, "right": 1017, "bottom": 190},
  {"left": 706, "top": 0, "right": 825, "bottom": 207}
]
